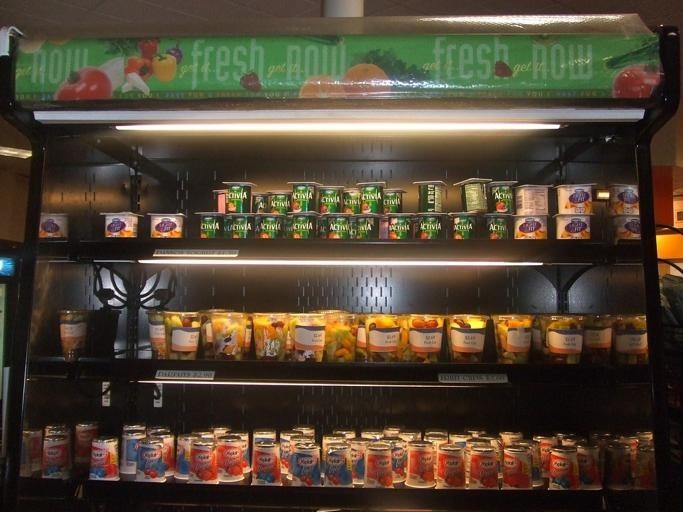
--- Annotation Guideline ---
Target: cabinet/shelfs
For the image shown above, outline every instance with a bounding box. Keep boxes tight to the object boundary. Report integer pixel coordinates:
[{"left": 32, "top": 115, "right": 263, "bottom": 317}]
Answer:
[{"left": 0, "top": 13, "right": 681, "bottom": 512}]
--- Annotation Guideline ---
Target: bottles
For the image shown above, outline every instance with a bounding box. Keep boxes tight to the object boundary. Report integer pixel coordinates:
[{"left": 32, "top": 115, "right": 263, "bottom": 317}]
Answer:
[
  {"left": 463, "top": 427, "right": 487, "bottom": 441},
  {"left": 216, "top": 435, "right": 245, "bottom": 482},
  {"left": 498, "top": 430, "right": 523, "bottom": 447},
  {"left": 41, "top": 435, "right": 71, "bottom": 479},
  {"left": 286, "top": 435, "right": 314, "bottom": 479},
  {"left": 422, "top": 434, "right": 448, "bottom": 480},
  {"left": 187, "top": 439, "right": 220, "bottom": 484},
  {"left": 23, "top": 428, "right": 42, "bottom": 471},
  {"left": 44, "top": 423, "right": 71, "bottom": 439},
  {"left": 465, "top": 439, "right": 491, "bottom": 484},
  {"left": 134, "top": 437, "right": 166, "bottom": 483},
  {"left": 404, "top": 440, "right": 436, "bottom": 488},
  {"left": 362, "top": 442, "right": 395, "bottom": 488},
  {"left": 173, "top": 434, "right": 201, "bottom": 479},
  {"left": 250, "top": 442, "right": 283, "bottom": 486},
  {"left": 291, "top": 443, "right": 322, "bottom": 487},
  {"left": 604, "top": 443, "right": 631, "bottom": 490},
  {"left": 322, "top": 443, "right": 355, "bottom": 488},
  {"left": 88, "top": 436, "right": 120, "bottom": 480},
  {"left": 561, "top": 436, "right": 587, "bottom": 447},
  {"left": 532, "top": 434, "right": 557, "bottom": 479},
  {"left": 122, "top": 421, "right": 146, "bottom": 431},
  {"left": 573, "top": 441, "right": 603, "bottom": 491},
  {"left": 225, "top": 430, "right": 251, "bottom": 474},
  {"left": 547, "top": 445, "right": 580, "bottom": 491},
  {"left": 73, "top": 420, "right": 98, "bottom": 466},
  {"left": 333, "top": 428, "right": 356, "bottom": 439},
  {"left": 150, "top": 432, "right": 174, "bottom": 476},
  {"left": 501, "top": 446, "right": 533, "bottom": 490},
  {"left": 633, "top": 431, "right": 656, "bottom": 490},
  {"left": 469, "top": 445, "right": 499, "bottom": 490},
  {"left": 383, "top": 425, "right": 402, "bottom": 440},
  {"left": 147, "top": 424, "right": 170, "bottom": 435},
  {"left": 251, "top": 428, "right": 277, "bottom": 468},
  {"left": 380, "top": 436, "right": 406, "bottom": 483},
  {"left": 361, "top": 429, "right": 384, "bottom": 440},
  {"left": 398, "top": 433, "right": 416, "bottom": 473},
  {"left": 120, "top": 430, "right": 146, "bottom": 475},
  {"left": 514, "top": 439, "right": 544, "bottom": 487},
  {"left": 320, "top": 433, "right": 346, "bottom": 477},
  {"left": 435, "top": 445, "right": 466, "bottom": 489},
  {"left": 292, "top": 423, "right": 316, "bottom": 443},
  {"left": 279, "top": 430, "right": 303, "bottom": 473},
  {"left": 212, "top": 426, "right": 232, "bottom": 436},
  {"left": 190, "top": 430, "right": 214, "bottom": 438},
  {"left": 424, "top": 428, "right": 448, "bottom": 436},
  {"left": 478, "top": 434, "right": 504, "bottom": 479},
  {"left": 350, "top": 440, "right": 369, "bottom": 484},
  {"left": 449, "top": 432, "right": 472, "bottom": 455}
]
